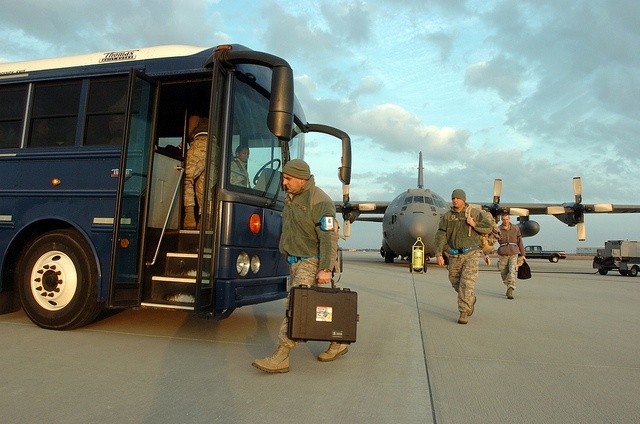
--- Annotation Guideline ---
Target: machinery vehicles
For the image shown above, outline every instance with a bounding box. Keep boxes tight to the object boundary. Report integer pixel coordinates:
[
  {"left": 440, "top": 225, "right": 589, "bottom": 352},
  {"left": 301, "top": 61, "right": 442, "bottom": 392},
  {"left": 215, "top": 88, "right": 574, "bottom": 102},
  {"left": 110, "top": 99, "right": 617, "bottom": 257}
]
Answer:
[{"left": 592, "top": 239, "right": 639, "bottom": 274}]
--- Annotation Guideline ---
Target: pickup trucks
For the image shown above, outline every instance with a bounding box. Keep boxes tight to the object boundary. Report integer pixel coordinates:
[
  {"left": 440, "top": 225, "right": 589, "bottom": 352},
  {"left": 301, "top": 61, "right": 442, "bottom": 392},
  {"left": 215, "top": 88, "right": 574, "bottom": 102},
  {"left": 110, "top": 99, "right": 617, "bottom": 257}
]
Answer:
[{"left": 522, "top": 245, "right": 565, "bottom": 262}]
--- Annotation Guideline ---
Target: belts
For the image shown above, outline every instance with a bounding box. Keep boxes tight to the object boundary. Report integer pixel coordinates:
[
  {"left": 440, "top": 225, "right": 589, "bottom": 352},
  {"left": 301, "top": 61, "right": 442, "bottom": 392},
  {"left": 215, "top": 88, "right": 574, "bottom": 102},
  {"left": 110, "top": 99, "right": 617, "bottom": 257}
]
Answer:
[{"left": 195, "top": 134, "right": 208, "bottom": 138}]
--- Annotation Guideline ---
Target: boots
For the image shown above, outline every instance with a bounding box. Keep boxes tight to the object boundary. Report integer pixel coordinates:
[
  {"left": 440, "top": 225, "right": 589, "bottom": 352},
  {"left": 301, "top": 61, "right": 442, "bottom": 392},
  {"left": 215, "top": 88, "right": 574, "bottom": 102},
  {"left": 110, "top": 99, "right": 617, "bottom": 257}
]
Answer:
[
  {"left": 507, "top": 287, "right": 516, "bottom": 299},
  {"left": 180, "top": 205, "right": 196, "bottom": 229},
  {"left": 317, "top": 341, "right": 349, "bottom": 362},
  {"left": 251, "top": 342, "right": 291, "bottom": 374},
  {"left": 469, "top": 295, "right": 476, "bottom": 316},
  {"left": 197, "top": 214, "right": 210, "bottom": 230},
  {"left": 458, "top": 312, "right": 469, "bottom": 324}
]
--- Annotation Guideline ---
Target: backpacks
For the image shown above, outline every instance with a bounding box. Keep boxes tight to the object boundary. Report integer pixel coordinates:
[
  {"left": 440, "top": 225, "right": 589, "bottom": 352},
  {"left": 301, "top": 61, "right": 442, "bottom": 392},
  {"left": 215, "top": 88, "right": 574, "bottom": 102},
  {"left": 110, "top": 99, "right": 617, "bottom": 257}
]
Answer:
[{"left": 465, "top": 203, "right": 503, "bottom": 246}]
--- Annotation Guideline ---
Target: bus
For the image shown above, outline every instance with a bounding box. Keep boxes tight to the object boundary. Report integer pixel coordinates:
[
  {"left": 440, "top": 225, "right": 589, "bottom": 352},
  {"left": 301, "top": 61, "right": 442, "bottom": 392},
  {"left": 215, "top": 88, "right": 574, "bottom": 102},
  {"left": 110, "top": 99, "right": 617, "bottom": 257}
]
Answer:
[{"left": 0, "top": 43, "right": 351, "bottom": 328}]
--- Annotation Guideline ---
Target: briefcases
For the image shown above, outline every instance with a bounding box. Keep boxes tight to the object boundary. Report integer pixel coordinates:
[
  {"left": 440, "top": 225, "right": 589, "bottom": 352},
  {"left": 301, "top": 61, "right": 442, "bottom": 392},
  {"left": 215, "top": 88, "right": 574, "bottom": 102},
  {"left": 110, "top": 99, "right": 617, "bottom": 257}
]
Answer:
[{"left": 286, "top": 278, "right": 359, "bottom": 344}]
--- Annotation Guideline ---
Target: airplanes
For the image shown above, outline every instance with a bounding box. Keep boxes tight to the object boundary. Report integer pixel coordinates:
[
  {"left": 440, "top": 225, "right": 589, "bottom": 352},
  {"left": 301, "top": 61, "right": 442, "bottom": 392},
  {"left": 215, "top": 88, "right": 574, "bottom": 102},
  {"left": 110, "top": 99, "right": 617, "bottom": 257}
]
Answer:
[{"left": 334, "top": 151, "right": 640, "bottom": 261}]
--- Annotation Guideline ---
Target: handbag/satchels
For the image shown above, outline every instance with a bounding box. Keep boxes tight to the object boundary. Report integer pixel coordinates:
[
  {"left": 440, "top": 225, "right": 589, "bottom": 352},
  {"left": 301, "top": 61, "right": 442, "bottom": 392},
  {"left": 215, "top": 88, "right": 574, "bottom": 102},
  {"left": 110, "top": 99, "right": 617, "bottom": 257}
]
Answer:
[{"left": 518, "top": 258, "right": 531, "bottom": 279}]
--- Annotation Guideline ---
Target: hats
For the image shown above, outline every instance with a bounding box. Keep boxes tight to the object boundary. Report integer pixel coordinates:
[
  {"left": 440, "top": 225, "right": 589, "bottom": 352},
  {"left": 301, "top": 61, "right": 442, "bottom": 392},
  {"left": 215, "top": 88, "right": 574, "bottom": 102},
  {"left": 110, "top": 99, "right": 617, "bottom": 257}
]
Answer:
[
  {"left": 282, "top": 159, "right": 311, "bottom": 181},
  {"left": 452, "top": 189, "right": 466, "bottom": 201}
]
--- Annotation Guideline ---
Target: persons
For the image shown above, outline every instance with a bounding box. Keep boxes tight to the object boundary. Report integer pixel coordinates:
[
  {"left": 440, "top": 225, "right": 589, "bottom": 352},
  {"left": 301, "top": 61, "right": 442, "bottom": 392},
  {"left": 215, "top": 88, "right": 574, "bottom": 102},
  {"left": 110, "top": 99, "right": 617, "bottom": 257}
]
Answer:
[
  {"left": 494, "top": 213, "right": 527, "bottom": 300},
  {"left": 435, "top": 188, "right": 493, "bottom": 324},
  {"left": 231, "top": 146, "right": 250, "bottom": 187},
  {"left": 105, "top": 91, "right": 158, "bottom": 152},
  {"left": 32, "top": 119, "right": 53, "bottom": 146},
  {"left": 180, "top": 114, "right": 219, "bottom": 231},
  {"left": 251, "top": 159, "right": 359, "bottom": 374}
]
